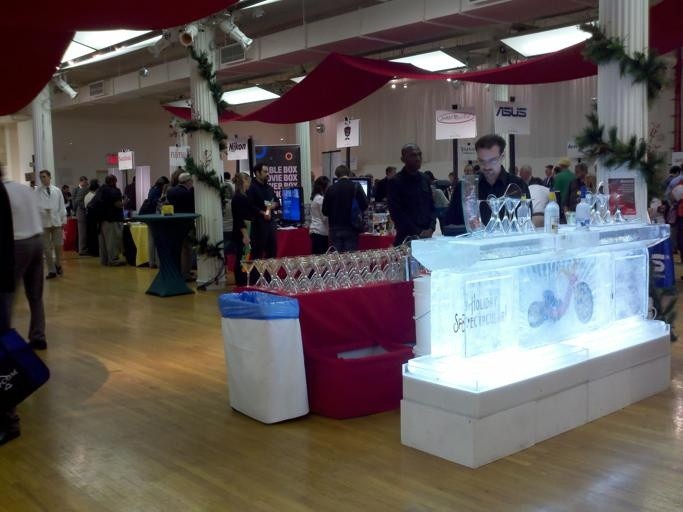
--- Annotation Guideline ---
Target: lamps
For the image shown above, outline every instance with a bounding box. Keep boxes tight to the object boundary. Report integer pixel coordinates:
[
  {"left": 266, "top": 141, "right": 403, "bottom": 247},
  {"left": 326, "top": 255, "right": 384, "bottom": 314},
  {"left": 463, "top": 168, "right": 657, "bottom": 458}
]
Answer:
[
  {"left": 290, "top": 75, "right": 307, "bottom": 84},
  {"left": 496, "top": 17, "right": 599, "bottom": 59},
  {"left": 147, "top": 18, "right": 254, "bottom": 58},
  {"left": 384, "top": 47, "right": 468, "bottom": 73},
  {"left": 54, "top": 75, "right": 79, "bottom": 101},
  {"left": 166, "top": 86, "right": 281, "bottom": 106},
  {"left": 139, "top": 68, "right": 148, "bottom": 77}
]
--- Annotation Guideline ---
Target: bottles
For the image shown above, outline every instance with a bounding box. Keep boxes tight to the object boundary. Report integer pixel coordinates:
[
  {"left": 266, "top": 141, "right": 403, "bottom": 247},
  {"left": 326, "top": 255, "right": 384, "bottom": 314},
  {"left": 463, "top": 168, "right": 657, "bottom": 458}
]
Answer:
[
  {"left": 543, "top": 191, "right": 561, "bottom": 233},
  {"left": 464, "top": 159, "right": 475, "bottom": 175},
  {"left": 572, "top": 186, "right": 589, "bottom": 231},
  {"left": 518, "top": 191, "right": 531, "bottom": 218}
]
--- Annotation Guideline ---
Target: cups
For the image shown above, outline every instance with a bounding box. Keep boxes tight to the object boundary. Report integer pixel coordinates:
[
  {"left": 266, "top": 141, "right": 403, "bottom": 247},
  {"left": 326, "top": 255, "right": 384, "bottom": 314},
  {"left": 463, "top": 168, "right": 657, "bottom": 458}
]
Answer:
[{"left": 565, "top": 211, "right": 575, "bottom": 225}]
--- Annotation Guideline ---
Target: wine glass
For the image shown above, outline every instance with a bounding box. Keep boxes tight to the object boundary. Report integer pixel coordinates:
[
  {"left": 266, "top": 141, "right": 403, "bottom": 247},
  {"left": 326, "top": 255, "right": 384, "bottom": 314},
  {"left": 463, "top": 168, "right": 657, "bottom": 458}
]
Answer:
[
  {"left": 481, "top": 196, "right": 535, "bottom": 234},
  {"left": 588, "top": 192, "right": 625, "bottom": 225},
  {"left": 237, "top": 245, "right": 429, "bottom": 295}
]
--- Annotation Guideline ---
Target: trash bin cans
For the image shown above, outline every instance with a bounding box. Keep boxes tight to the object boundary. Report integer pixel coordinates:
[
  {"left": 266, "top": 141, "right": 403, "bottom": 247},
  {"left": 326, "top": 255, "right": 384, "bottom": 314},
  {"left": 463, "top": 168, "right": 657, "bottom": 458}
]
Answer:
[
  {"left": 218, "top": 291, "right": 310, "bottom": 424},
  {"left": 252, "top": 144, "right": 302, "bottom": 219}
]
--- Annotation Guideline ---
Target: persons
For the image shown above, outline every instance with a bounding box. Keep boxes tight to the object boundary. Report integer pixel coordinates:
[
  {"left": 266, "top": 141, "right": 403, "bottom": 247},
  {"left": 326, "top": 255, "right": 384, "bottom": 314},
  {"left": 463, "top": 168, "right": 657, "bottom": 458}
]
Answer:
[
  {"left": 33, "top": 169, "right": 68, "bottom": 278},
  {"left": 62, "top": 168, "right": 194, "bottom": 266},
  {"left": 308, "top": 165, "right": 398, "bottom": 254},
  {"left": 440, "top": 132, "right": 533, "bottom": 234},
  {"left": 219, "top": 164, "right": 282, "bottom": 286},
  {"left": 383, "top": 141, "right": 437, "bottom": 246},
  {"left": 423, "top": 163, "right": 480, "bottom": 227},
  {"left": 661, "top": 165, "right": 681, "bottom": 225},
  {"left": 0, "top": 168, "right": 13, "bottom": 338},
  {"left": 0, "top": 180, "right": 47, "bottom": 351},
  {"left": 519, "top": 160, "right": 589, "bottom": 211}
]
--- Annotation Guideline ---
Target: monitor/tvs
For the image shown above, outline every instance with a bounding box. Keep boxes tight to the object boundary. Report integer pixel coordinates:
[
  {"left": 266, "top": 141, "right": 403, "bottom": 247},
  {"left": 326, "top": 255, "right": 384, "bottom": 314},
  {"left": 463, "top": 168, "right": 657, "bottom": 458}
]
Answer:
[
  {"left": 333, "top": 177, "right": 371, "bottom": 202},
  {"left": 280, "top": 186, "right": 305, "bottom": 226}
]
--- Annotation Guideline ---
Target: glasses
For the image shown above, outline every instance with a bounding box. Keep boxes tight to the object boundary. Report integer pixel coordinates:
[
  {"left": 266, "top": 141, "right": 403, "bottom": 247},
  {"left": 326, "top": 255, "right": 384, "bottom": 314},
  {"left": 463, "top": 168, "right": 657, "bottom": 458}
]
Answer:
[{"left": 478, "top": 154, "right": 501, "bottom": 164}]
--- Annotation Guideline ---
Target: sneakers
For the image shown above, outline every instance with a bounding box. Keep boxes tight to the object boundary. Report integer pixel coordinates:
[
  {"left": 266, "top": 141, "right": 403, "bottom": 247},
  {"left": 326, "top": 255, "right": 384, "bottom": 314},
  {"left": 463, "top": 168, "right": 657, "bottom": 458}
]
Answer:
[
  {"left": 55, "top": 262, "right": 62, "bottom": 274},
  {"left": 27, "top": 339, "right": 48, "bottom": 351},
  {"left": 46, "top": 271, "right": 56, "bottom": 280}
]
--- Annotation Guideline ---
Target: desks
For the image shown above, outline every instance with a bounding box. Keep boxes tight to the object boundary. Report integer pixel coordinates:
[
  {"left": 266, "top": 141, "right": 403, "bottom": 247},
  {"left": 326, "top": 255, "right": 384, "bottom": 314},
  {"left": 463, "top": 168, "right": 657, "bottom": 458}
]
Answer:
[
  {"left": 132, "top": 213, "right": 201, "bottom": 297},
  {"left": 358, "top": 231, "right": 395, "bottom": 252},
  {"left": 274, "top": 227, "right": 311, "bottom": 259},
  {"left": 62, "top": 216, "right": 79, "bottom": 253},
  {"left": 123, "top": 221, "right": 150, "bottom": 267},
  {"left": 233, "top": 267, "right": 427, "bottom": 420}
]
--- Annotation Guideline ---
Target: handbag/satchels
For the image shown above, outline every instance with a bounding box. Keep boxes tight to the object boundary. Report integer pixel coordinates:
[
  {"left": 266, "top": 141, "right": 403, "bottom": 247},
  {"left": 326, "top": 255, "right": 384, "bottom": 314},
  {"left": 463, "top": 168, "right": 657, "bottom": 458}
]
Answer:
[
  {"left": 351, "top": 182, "right": 364, "bottom": 229},
  {"left": 0, "top": 328, "right": 50, "bottom": 412}
]
